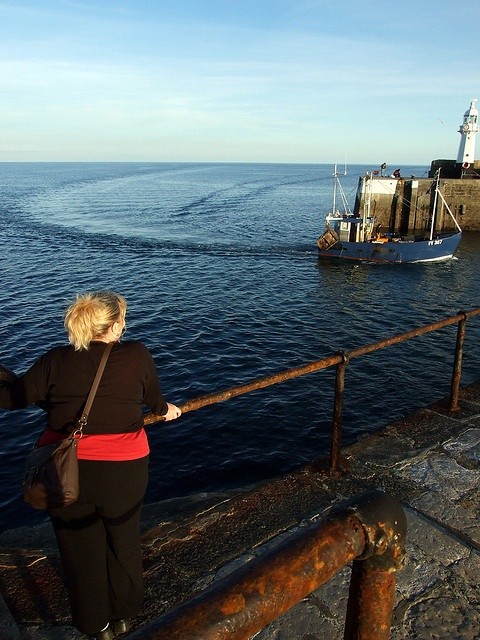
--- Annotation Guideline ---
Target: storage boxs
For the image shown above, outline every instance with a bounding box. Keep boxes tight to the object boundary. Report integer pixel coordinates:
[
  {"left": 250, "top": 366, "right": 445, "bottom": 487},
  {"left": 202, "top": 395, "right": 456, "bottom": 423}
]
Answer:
[{"left": 317, "top": 229, "right": 340, "bottom": 252}]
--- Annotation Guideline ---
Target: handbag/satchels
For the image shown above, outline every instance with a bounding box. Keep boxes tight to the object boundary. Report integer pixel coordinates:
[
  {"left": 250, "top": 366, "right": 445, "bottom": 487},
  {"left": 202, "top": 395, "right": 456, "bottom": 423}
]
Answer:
[{"left": 22, "top": 436, "right": 81, "bottom": 510}]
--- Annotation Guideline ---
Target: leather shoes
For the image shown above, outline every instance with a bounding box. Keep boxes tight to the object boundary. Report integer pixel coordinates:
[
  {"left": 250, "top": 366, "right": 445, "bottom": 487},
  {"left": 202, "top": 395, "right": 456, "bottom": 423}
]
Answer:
[
  {"left": 96, "top": 626, "right": 116, "bottom": 640},
  {"left": 112, "top": 617, "right": 132, "bottom": 634}
]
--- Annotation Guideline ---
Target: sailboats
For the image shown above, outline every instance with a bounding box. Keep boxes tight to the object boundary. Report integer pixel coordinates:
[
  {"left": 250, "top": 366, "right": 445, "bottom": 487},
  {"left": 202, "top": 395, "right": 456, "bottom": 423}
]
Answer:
[{"left": 317, "top": 164, "right": 462, "bottom": 265}]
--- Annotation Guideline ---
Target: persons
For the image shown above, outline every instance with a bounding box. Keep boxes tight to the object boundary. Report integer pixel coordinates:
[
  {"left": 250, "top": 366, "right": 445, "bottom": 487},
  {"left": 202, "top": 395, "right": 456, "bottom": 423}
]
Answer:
[
  {"left": 1, "top": 290, "right": 181, "bottom": 639},
  {"left": 381, "top": 163, "right": 386, "bottom": 177},
  {"left": 394, "top": 169, "right": 401, "bottom": 177},
  {"left": 376, "top": 223, "right": 381, "bottom": 241}
]
